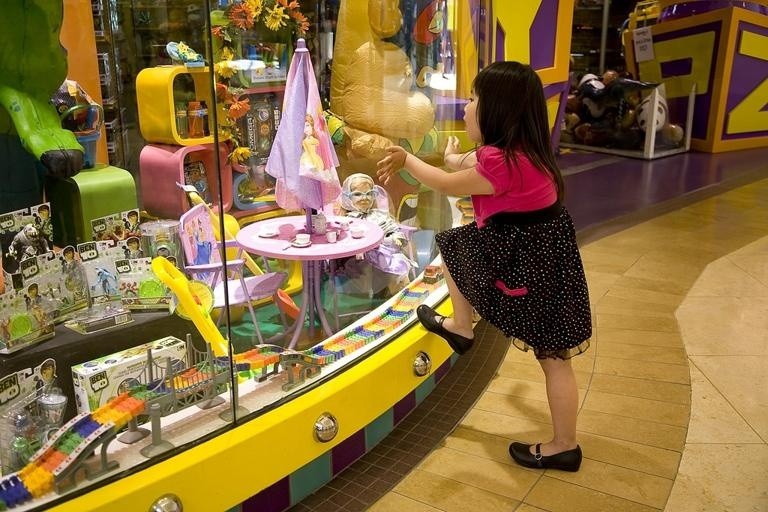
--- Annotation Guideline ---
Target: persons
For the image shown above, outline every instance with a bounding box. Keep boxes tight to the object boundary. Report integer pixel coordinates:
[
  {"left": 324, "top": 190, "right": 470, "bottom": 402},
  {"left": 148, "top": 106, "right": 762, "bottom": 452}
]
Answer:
[
  {"left": 185, "top": 220, "right": 221, "bottom": 279},
  {"left": 328, "top": 172, "right": 413, "bottom": 294},
  {"left": 20, "top": 201, "right": 178, "bottom": 391},
  {"left": 296, "top": 118, "right": 323, "bottom": 176},
  {"left": 373, "top": 60, "right": 593, "bottom": 472}
]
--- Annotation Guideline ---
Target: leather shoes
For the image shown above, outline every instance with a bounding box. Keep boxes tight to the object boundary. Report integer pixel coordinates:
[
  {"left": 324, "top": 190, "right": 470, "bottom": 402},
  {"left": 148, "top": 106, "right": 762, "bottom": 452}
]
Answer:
[
  {"left": 417, "top": 304, "right": 474, "bottom": 355},
  {"left": 510, "top": 442, "right": 582, "bottom": 471}
]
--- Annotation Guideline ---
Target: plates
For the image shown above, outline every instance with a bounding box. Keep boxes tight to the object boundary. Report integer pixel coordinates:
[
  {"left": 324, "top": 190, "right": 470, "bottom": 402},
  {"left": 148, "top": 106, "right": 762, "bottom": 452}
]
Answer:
[
  {"left": 291, "top": 240, "right": 312, "bottom": 248},
  {"left": 259, "top": 231, "right": 277, "bottom": 238}
]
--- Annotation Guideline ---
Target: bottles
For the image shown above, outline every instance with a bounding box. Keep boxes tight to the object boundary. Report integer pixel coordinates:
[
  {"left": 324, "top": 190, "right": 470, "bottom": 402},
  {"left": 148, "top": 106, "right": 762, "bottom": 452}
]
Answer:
[
  {"left": 314, "top": 212, "right": 326, "bottom": 235},
  {"left": 175, "top": 100, "right": 210, "bottom": 139}
]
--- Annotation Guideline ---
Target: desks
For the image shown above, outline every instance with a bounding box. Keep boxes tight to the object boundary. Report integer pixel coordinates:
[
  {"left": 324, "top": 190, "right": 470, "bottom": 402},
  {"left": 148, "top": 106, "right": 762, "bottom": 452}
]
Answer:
[{"left": 234, "top": 216, "right": 383, "bottom": 350}]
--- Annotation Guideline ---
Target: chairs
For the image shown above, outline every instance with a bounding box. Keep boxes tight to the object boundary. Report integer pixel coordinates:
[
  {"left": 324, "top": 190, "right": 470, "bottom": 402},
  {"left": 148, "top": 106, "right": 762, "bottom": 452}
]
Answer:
[
  {"left": 179, "top": 203, "right": 286, "bottom": 348},
  {"left": 341, "top": 185, "right": 418, "bottom": 300}
]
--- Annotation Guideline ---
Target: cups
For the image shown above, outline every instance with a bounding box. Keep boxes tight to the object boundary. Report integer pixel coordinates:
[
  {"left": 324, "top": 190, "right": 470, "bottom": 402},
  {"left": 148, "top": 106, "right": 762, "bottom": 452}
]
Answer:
[
  {"left": 350, "top": 227, "right": 363, "bottom": 239},
  {"left": 326, "top": 232, "right": 337, "bottom": 243},
  {"left": 38, "top": 395, "right": 69, "bottom": 427},
  {"left": 296, "top": 234, "right": 310, "bottom": 244}
]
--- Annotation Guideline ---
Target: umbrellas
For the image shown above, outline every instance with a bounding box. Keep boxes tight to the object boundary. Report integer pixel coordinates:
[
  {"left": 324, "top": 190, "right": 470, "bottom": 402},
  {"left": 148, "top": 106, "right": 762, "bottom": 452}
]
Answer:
[{"left": 266, "top": 39, "right": 344, "bottom": 239}]
